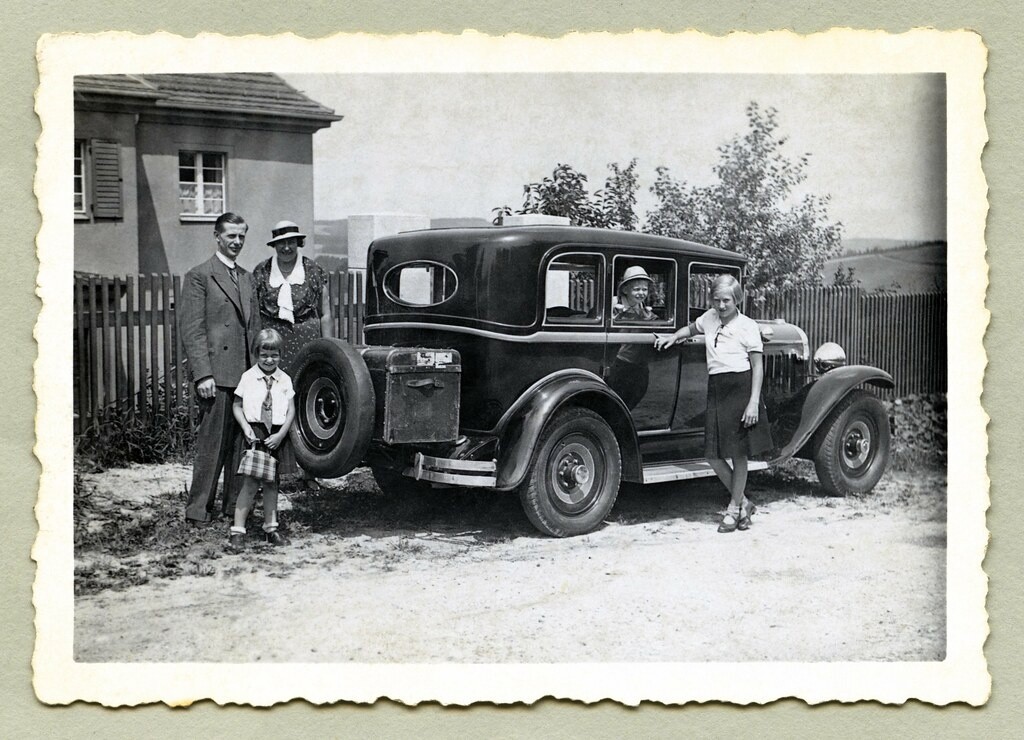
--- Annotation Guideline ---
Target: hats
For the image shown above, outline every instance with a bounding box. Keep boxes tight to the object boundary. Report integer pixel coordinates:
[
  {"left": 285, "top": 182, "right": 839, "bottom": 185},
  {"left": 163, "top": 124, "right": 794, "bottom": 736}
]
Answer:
[
  {"left": 267, "top": 221, "right": 306, "bottom": 248},
  {"left": 618, "top": 265, "right": 653, "bottom": 295}
]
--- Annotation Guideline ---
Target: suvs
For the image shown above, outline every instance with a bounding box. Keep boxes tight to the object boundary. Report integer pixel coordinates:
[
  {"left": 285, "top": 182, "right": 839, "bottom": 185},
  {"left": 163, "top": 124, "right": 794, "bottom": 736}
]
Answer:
[{"left": 285, "top": 211, "right": 899, "bottom": 540}]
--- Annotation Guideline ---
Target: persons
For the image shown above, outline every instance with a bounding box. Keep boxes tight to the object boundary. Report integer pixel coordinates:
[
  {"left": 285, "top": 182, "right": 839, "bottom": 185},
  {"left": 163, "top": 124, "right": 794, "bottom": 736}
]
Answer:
[
  {"left": 596, "top": 327, "right": 653, "bottom": 415},
  {"left": 229, "top": 326, "right": 297, "bottom": 550},
  {"left": 181, "top": 210, "right": 263, "bottom": 526},
  {"left": 613, "top": 266, "right": 661, "bottom": 321},
  {"left": 253, "top": 220, "right": 333, "bottom": 490},
  {"left": 652, "top": 274, "right": 775, "bottom": 533}
]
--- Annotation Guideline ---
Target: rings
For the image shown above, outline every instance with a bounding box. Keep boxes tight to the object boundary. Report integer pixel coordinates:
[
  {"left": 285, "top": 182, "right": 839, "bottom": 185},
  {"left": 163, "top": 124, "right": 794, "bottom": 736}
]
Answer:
[{"left": 752, "top": 417, "right": 756, "bottom": 421}]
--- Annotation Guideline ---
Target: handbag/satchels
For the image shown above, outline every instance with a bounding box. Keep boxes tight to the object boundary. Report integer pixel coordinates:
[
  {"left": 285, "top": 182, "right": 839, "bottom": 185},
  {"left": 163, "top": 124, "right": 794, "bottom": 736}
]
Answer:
[{"left": 236, "top": 439, "right": 278, "bottom": 482}]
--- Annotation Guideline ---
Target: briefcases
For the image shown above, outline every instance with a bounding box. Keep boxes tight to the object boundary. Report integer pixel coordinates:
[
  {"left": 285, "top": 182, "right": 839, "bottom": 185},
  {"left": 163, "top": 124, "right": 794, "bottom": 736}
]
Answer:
[{"left": 355, "top": 345, "right": 462, "bottom": 446}]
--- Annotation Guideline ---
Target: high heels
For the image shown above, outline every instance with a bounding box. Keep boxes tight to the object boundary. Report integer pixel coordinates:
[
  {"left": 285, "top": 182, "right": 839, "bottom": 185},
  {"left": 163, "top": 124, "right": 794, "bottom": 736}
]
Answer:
[
  {"left": 739, "top": 500, "right": 757, "bottom": 530},
  {"left": 718, "top": 509, "right": 741, "bottom": 533}
]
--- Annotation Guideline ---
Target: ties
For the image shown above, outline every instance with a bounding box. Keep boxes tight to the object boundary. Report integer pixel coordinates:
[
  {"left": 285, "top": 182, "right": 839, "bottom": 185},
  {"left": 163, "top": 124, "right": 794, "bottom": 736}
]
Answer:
[{"left": 261, "top": 376, "right": 274, "bottom": 435}]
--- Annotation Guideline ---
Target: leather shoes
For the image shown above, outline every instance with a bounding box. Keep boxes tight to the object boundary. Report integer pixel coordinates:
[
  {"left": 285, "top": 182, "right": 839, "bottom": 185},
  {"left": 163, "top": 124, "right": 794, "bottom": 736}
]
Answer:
[
  {"left": 185, "top": 518, "right": 211, "bottom": 528},
  {"left": 230, "top": 535, "right": 246, "bottom": 552},
  {"left": 264, "top": 530, "right": 291, "bottom": 546}
]
